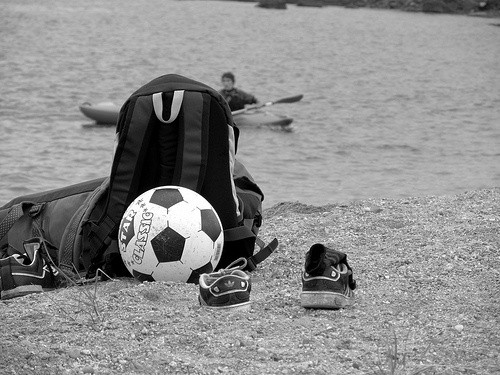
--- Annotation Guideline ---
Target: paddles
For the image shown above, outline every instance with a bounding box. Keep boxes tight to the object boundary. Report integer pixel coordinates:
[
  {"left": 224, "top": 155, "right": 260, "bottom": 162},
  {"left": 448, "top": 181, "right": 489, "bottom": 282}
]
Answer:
[{"left": 231, "top": 94, "right": 303, "bottom": 116}]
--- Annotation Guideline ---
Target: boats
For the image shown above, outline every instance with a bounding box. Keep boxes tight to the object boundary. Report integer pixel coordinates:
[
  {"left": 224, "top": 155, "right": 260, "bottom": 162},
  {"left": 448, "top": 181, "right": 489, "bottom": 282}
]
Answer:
[{"left": 79, "top": 100, "right": 294, "bottom": 129}]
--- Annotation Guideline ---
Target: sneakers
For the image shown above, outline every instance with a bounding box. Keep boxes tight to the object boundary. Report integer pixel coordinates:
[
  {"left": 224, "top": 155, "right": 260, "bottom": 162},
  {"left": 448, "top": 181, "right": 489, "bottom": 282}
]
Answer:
[
  {"left": 0, "top": 251, "right": 57, "bottom": 299},
  {"left": 300, "top": 243, "right": 357, "bottom": 308},
  {"left": 199, "top": 257, "right": 251, "bottom": 315}
]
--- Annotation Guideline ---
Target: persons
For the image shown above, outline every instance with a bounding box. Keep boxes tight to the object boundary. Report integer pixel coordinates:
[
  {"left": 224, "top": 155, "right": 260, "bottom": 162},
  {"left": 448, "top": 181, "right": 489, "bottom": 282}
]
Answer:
[{"left": 213, "top": 73, "right": 259, "bottom": 118}]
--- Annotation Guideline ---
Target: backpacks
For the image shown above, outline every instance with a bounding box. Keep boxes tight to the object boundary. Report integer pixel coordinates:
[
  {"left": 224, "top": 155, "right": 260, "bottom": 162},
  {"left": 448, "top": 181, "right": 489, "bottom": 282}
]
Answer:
[{"left": 83, "top": 73, "right": 280, "bottom": 279}]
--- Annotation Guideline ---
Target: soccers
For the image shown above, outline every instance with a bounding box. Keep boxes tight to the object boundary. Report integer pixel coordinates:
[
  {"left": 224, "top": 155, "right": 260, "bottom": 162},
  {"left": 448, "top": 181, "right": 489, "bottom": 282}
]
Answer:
[{"left": 118, "top": 185, "right": 224, "bottom": 285}]
